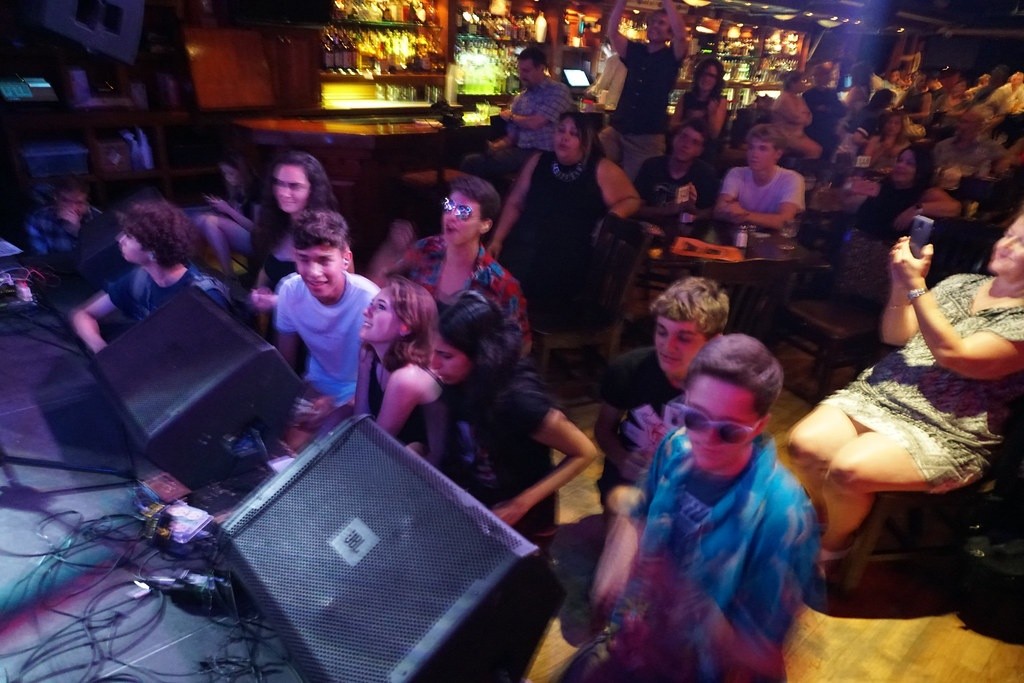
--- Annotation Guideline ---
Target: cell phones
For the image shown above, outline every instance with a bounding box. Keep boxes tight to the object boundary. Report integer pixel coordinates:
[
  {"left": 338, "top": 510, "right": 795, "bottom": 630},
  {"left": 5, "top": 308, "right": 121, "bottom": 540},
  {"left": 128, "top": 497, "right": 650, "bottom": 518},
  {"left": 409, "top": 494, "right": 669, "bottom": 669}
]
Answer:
[{"left": 908, "top": 216, "right": 934, "bottom": 259}]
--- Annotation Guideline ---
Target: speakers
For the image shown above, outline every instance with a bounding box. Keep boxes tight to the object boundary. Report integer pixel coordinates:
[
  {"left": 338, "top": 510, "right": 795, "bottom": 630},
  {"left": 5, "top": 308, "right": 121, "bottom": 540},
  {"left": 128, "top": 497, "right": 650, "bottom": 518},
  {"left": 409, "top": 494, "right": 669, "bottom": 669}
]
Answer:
[
  {"left": 77, "top": 187, "right": 173, "bottom": 291},
  {"left": 216, "top": 411, "right": 567, "bottom": 683},
  {"left": 11, "top": 0, "right": 145, "bottom": 66},
  {"left": 89, "top": 283, "right": 309, "bottom": 492}
]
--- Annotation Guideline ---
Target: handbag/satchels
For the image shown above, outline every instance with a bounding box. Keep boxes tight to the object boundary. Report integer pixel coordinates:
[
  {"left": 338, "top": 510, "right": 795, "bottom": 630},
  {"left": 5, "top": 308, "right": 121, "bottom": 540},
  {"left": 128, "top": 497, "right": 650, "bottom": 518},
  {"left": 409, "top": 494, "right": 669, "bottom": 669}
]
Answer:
[{"left": 941, "top": 526, "right": 1024, "bottom": 646}]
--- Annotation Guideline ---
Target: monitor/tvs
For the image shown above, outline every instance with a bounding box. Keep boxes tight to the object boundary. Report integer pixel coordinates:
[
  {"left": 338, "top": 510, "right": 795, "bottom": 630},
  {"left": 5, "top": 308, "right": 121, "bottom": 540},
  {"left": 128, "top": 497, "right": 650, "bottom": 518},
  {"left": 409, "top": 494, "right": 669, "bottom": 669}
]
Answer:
[
  {"left": 227, "top": 0, "right": 334, "bottom": 29},
  {"left": 563, "top": 69, "right": 591, "bottom": 88}
]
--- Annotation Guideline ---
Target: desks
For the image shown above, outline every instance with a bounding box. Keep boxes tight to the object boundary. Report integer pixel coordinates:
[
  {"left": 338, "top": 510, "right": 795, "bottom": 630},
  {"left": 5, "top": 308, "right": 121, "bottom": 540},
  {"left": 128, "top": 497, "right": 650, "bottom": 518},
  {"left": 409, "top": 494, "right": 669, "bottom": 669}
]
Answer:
[{"left": 647, "top": 213, "right": 833, "bottom": 342}]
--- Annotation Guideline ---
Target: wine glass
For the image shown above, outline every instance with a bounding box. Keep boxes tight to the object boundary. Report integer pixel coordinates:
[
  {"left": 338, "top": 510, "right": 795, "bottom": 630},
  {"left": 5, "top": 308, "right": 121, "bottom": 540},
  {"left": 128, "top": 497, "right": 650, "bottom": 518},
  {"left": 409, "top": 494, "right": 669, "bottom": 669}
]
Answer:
[{"left": 778, "top": 218, "right": 801, "bottom": 250}]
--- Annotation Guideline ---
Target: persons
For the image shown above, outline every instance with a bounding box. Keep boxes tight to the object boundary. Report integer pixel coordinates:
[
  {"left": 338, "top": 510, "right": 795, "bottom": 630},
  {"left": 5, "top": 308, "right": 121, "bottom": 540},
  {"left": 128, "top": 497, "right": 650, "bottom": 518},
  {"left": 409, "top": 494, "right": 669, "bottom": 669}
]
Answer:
[
  {"left": 598, "top": 0, "right": 686, "bottom": 184},
  {"left": 786, "top": 203, "right": 1024, "bottom": 581},
  {"left": 29, "top": 48, "right": 1024, "bottom": 527},
  {"left": 590, "top": 279, "right": 732, "bottom": 627},
  {"left": 557, "top": 333, "right": 819, "bottom": 683}
]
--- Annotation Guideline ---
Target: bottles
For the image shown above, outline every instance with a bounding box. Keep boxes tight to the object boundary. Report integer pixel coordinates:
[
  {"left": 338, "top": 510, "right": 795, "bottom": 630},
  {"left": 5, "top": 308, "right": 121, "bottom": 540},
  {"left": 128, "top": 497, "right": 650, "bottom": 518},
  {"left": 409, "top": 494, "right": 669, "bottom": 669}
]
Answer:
[
  {"left": 735, "top": 223, "right": 749, "bottom": 247},
  {"left": 320, "top": 1, "right": 830, "bottom": 123}
]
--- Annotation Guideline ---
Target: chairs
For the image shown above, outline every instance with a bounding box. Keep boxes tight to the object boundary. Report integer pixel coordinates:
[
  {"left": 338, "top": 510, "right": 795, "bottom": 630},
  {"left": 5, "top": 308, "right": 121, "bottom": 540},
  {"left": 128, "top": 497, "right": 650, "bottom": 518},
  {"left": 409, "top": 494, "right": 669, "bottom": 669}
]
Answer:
[
  {"left": 782, "top": 201, "right": 933, "bottom": 384},
  {"left": 524, "top": 213, "right": 652, "bottom": 383}
]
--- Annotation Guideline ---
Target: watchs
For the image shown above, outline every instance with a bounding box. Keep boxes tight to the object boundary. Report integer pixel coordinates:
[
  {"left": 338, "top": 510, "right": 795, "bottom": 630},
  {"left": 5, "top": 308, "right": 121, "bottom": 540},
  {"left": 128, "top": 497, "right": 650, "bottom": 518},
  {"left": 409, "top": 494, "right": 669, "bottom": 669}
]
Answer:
[{"left": 906, "top": 287, "right": 927, "bottom": 304}]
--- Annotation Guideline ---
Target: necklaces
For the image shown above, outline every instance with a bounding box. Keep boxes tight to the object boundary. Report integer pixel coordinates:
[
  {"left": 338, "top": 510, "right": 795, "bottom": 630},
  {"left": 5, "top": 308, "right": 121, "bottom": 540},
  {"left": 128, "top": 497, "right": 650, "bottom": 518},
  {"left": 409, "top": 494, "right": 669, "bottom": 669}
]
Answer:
[{"left": 552, "top": 158, "right": 586, "bottom": 182}]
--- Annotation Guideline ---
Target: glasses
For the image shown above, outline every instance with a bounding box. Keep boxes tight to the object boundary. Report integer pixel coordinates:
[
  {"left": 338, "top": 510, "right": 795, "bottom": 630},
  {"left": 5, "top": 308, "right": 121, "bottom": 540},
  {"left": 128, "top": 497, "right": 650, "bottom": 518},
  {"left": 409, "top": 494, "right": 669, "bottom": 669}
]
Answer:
[
  {"left": 681, "top": 403, "right": 762, "bottom": 445},
  {"left": 700, "top": 71, "right": 717, "bottom": 80},
  {"left": 441, "top": 199, "right": 488, "bottom": 222}
]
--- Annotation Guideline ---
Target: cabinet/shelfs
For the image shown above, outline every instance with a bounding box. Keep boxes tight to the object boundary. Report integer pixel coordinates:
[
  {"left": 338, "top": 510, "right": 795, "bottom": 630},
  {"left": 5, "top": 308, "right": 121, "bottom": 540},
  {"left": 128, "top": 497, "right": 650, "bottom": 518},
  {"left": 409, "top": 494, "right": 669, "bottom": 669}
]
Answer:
[
  {"left": 280, "top": 0, "right": 803, "bottom": 116},
  {"left": 6, "top": 93, "right": 224, "bottom": 234}
]
